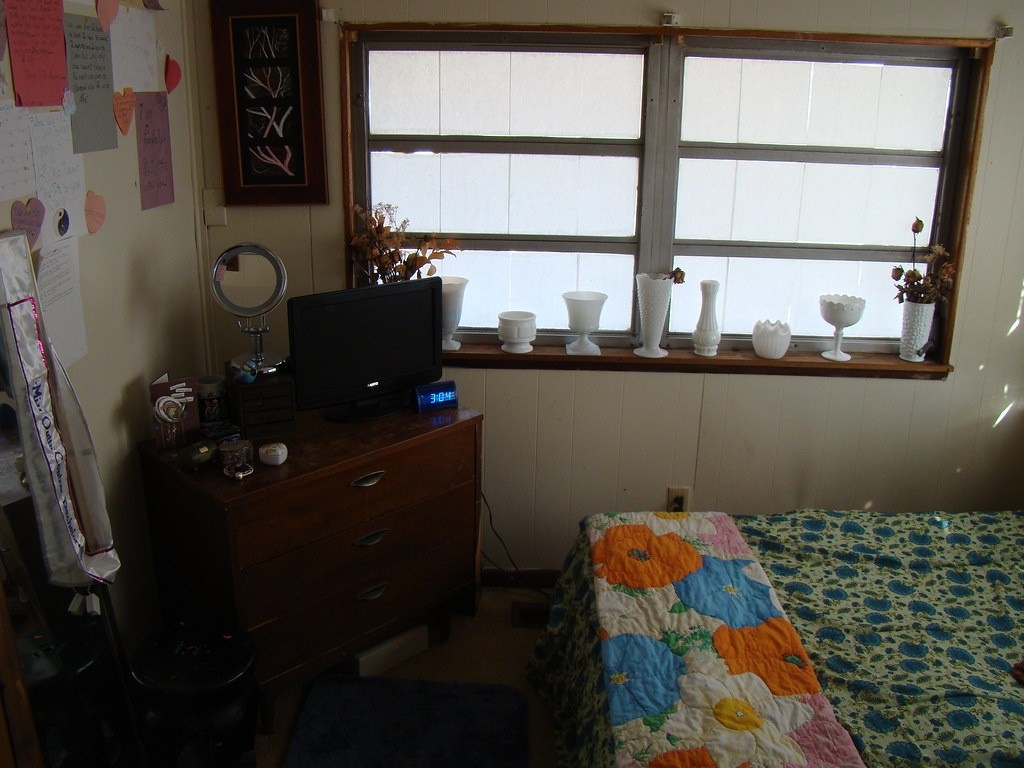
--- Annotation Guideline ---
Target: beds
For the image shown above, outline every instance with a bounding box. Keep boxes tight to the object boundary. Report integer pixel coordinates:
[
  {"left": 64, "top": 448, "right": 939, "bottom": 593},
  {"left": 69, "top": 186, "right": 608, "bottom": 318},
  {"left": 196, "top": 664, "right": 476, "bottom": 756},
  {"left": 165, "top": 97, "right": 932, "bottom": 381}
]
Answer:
[{"left": 538, "top": 508, "right": 1024, "bottom": 768}]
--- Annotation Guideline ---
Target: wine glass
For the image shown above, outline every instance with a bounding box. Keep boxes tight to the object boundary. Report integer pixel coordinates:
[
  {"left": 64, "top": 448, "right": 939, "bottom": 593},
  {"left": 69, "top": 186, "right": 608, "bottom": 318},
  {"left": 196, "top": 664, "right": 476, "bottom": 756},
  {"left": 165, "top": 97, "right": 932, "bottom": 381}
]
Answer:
[
  {"left": 819, "top": 294, "right": 866, "bottom": 362},
  {"left": 440, "top": 276, "right": 469, "bottom": 350},
  {"left": 562, "top": 291, "right": 608, "bottom": 357}
]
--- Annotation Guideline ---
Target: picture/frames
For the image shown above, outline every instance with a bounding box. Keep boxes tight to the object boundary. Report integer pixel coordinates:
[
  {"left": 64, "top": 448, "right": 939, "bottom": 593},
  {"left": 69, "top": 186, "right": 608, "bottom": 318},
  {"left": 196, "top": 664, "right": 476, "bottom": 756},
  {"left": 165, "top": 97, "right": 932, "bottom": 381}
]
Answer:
[{"left": 208, "top": 0, "right": 330, "bottom": 208}]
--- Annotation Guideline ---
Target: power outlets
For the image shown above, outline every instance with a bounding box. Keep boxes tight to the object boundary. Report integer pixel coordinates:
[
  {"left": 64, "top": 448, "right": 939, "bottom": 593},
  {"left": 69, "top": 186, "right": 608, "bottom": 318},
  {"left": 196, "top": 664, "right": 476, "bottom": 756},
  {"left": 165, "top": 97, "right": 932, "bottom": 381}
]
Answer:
[{"left": 667, "top": 486, "right": 689, "bottom": 513}]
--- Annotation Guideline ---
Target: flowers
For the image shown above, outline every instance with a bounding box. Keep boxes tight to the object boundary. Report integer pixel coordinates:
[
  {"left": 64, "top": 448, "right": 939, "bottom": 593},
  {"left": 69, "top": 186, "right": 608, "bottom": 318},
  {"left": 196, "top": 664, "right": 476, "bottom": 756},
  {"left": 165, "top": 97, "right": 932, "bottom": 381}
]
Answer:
[
  {"left": 892, "top": 217, "right": 956, "bottom": 304},
  {"left": 349, "top": 200, "right": 458, "bottom": 286},
  {"left": 662, "top": 268, "right": 686, "bottom": 284}
]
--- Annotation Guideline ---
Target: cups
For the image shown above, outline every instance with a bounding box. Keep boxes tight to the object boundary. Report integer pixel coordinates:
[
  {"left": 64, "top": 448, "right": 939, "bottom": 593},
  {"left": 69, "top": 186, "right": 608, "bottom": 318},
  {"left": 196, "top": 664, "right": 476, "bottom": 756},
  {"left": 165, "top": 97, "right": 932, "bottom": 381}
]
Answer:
[
  {"left": 633, "top": 271, "right": 675, "bottom": 358},
  {"left": 751, "top": 320, "right": 792, "bottom": 359},
  {"left": 498, "top": 311, "right": 536, "bottom": 354}
]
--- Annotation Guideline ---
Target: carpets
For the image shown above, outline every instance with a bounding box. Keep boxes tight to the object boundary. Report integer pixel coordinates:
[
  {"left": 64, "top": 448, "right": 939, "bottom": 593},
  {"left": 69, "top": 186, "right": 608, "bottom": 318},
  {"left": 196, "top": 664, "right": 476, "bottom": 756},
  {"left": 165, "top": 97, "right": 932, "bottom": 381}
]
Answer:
[{"left": 281, "top": 671, "right": 532, "bottom": 767}]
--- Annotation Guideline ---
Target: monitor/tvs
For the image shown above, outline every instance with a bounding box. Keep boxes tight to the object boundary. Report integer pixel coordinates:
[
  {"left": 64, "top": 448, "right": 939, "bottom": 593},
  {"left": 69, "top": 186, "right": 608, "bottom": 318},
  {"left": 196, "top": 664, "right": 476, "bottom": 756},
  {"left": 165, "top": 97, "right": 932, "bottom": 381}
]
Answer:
[{"left": 286, "top": 277, "right": 444, "bottom": 423}]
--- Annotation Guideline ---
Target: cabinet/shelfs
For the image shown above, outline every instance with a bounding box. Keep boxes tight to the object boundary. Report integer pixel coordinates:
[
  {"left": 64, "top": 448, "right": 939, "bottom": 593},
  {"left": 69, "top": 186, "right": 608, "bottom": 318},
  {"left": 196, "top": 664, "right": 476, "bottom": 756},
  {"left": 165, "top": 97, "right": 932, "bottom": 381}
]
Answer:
[
  {"left": 136, "top": 398, "right": 484, "bottom": 735},
  {"left": 239, "top": 374, "right": 298, "bottom": 442}
]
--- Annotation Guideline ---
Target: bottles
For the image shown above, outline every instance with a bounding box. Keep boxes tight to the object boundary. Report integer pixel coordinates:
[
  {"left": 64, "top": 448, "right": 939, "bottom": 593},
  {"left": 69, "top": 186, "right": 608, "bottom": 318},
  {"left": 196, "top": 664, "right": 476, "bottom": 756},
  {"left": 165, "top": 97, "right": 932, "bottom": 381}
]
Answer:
[
  {"left": 691, "top": 280, "right": 721, "bottom": 356},
  {"left": 195, "top": 376, "right": 227, "bottom": 436}
]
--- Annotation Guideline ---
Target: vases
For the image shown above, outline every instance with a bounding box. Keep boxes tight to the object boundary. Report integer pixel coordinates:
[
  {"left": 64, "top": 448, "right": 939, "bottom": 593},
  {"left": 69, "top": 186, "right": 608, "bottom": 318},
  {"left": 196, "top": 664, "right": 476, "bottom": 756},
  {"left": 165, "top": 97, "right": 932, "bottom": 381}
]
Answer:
[
  {"left": 692, "top": 280, "right": 722, "bottom": 356},
  {"left": 440, "top": 276, "right": 470, "bottom": 351},
  {"left": 899, "top": 299, "right": 935, "bottom": 362},
  {"left": 564, "top": 290, "right": 608, "bottom": 355},
  {"left": 498, "top": 311, "right": 537, "bottom": 353},
  {"left": 633, "top": 273, "right": 668, "bottom": 358},
  {"left": 820, "top": 294, "right": 866, "bottom": 362},
  {"left": 753, "top": 320, "right": 791, "bottom": 359}
]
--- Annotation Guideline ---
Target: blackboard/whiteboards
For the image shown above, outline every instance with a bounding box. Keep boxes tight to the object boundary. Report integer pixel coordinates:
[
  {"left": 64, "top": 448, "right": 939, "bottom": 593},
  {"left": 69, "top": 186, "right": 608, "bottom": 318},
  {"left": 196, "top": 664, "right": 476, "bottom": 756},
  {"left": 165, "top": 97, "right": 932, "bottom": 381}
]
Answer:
[{"left": 0, "top": 0, "right": 225, "bottom": 544}]
